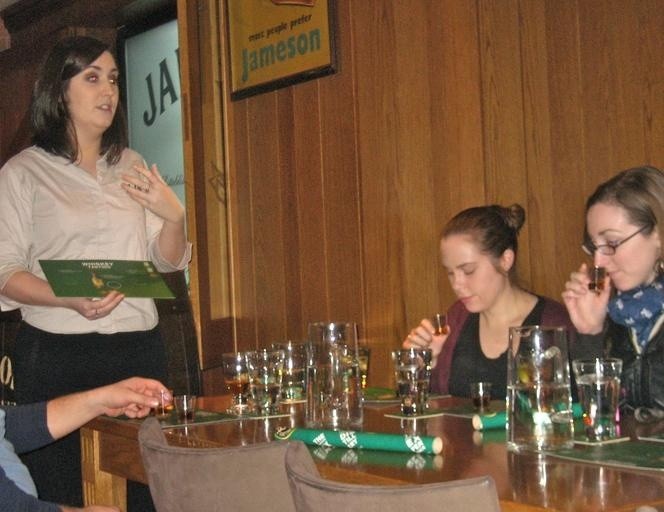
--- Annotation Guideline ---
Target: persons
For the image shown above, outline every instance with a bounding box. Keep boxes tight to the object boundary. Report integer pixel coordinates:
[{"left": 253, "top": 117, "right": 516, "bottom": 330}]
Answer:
[
  {"left": 398, "top": 204, "right": 580, "bottom": 402},
  {"left": 0, "top": 375, "right": 172, "bottom": 511},
  {"left": 562, "top": 164, "right": 664, "bottom": 418},
  {"left": 0, "top": 34, "right": 195, "bottom": 510}
]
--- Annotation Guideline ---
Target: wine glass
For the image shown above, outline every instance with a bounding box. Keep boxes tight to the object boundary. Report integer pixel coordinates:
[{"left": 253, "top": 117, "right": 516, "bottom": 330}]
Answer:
[{"left": 222, "top": 352, "right": 250, "bottom": 416}]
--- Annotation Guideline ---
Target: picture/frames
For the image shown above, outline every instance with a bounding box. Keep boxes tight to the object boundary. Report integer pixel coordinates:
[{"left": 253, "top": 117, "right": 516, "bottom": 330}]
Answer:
[{"left": 225, "top": 0, "right": 338, "bottom": 100}]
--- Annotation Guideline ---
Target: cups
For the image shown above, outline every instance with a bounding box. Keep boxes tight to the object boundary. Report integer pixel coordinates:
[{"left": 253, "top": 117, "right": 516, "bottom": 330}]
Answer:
[
  {"left": 470, "top": 382, "right": 492, "bottom": 415},
  {"left": 307, "top": 320, "right": 363, "bottom": 430},
  {"left": 174, "top": 396, "right": 195, "bottom": 423},
  {"left": 432, "top": 311, "right": 448, "bottom": 335},
  {"left": 587, "top": 267, "right": 606, "bottom": 290},
  {"left": 391, "top": 349, "right": 432, "bottom": 416},
  {"left": 507, "top": 326, "right": 574, "bottom": 456},
  {"left": 245, "top": 350, "right": 284, "bottom": 416},
  {"left": 272, "top": 343, "right": 307, "bottom": 402},
  {"left": 572, "top": 358, "right": 622, "bottom": 443},
  {"left": 152, "top": 391, "right": 174, "bottom": 417}
]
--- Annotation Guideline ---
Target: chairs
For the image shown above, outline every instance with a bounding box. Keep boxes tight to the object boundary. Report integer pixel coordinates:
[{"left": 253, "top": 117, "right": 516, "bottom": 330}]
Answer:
[
  {"left": 137, "top": 415, "right": 322, "bottom": 512},
  {"left": 197, "top": 362, "right": 247, "bottom": 397},
  {"left": 285, "top": 440, "right": 499, "bottom": 512}
]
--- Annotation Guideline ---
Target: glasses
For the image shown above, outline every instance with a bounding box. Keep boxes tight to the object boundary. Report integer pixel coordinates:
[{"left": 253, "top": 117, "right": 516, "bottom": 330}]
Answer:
[{"left": 580, "top": 227, "right": 643, "bottom": 256}]
